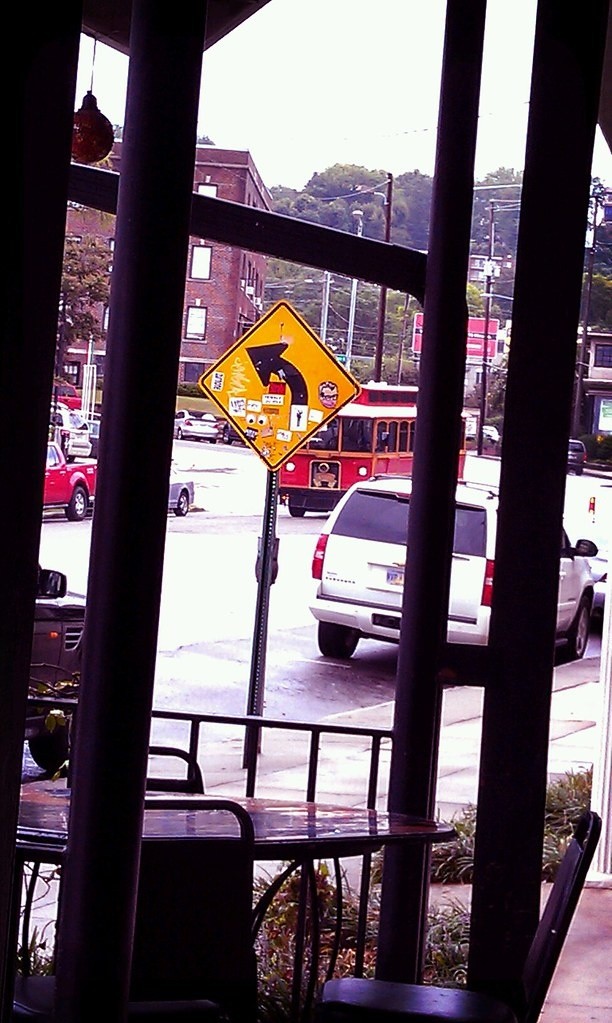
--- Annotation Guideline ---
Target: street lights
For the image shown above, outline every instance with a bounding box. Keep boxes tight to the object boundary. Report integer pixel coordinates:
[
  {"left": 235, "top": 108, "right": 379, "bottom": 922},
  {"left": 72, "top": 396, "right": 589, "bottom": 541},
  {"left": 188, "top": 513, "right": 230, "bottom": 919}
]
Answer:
[{"left": 342, "top": 210, "right": 365, "bottom": 371}]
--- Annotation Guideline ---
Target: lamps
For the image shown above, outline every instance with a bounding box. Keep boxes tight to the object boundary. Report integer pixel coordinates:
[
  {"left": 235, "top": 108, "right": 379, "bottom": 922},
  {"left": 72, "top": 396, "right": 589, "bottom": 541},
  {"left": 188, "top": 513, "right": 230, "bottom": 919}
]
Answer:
[
  {"left": 195, "top": 298, "right": 201, "bottom": 308},
  {"left": 200, "top": 239, "right": 205, "bottom": 246},
  {"left": 206, "top": 176, "right": 211, "bottom": 183}
]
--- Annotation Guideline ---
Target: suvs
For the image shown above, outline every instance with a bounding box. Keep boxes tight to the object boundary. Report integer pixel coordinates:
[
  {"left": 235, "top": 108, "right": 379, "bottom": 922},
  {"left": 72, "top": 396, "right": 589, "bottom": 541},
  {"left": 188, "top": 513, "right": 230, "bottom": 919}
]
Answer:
[
  {"left": 308, "top": 472, "right": 599, "bottom": 685},
  {"left": 566, "top": 438, "right": 589, "bottom": 476}
]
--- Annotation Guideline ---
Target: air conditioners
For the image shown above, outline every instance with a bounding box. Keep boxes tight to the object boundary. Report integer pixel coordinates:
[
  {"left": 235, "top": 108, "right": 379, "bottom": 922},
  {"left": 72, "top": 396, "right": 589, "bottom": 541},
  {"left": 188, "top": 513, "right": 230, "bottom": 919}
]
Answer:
[
  {"left": 241, "top": 279, "right": 245, "bottom": 287},
  {"left": 259, "top": 304, "right": 263, "bottom": 311},
  {"left": 255, "top": 297, "right": 261, "bottom": 305},
  {"left": 247, "top": 287, "right": 255, "bottom": 294}
]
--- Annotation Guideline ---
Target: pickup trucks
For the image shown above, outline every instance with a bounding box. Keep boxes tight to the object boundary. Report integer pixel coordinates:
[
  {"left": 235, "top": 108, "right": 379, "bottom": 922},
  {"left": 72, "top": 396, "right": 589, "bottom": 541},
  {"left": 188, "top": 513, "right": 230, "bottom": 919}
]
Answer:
[{"left": 42, "top": 441, "right": 98, "bottom": 522}]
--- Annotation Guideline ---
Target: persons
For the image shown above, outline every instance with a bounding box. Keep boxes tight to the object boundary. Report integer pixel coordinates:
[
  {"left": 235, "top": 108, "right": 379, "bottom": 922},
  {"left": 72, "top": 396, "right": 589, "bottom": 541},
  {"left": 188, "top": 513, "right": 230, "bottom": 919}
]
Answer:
[
  {"left": 330, "top": 420, "right": 337, "bottom": 431},
  {"left": 348, "top": 420, "right": 365, "bottom": 445}
]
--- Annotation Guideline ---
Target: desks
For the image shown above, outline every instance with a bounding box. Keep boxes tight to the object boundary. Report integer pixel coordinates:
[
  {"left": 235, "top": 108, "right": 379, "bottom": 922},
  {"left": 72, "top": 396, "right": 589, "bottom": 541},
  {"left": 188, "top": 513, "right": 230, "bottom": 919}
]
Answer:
[{"left": 16, "top": 784, "right": 458, "bottom": 1023}]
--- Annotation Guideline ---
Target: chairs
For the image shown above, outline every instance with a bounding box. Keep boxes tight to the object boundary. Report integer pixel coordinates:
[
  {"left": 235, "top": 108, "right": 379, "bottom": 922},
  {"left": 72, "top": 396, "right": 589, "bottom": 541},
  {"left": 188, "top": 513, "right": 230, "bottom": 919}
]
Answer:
[
  {"left": 12, "top": 797, "right": 258, "bottom": 1023},
  {"left": 321, "top": 811, "right": 601, "bottom": 1023},
  {"left": 146, "top": 748, "right": 206, "bottom": 793}
]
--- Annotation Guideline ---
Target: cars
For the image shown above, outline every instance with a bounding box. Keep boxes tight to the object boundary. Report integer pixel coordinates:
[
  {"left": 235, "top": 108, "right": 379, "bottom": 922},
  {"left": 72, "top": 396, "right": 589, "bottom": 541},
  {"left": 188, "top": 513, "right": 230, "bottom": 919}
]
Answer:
[
  {"left": 222, "top": 419, "right": 251, "bottom": 448},
  {"left": 48, "top": 376, "right": 100, "bottom": 463},
  {"left": 168, "top": 459, "right": 196, "bottom": 517},
  {"left": 172, "top": 409, "right": 220, "bottom": 444},
  {"left": 562, "top": 476, "right": 612, "bottom": 631},
  {"left": 23, "top": 564, "right": 88, "bottom": 771}
]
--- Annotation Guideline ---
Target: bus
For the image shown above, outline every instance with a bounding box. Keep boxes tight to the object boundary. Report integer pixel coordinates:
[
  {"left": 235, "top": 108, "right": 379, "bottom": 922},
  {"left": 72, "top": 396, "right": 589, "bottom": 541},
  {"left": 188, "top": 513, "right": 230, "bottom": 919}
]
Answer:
[{"left": 277, "top": 380, "right": 468, "bottom": 518}]
